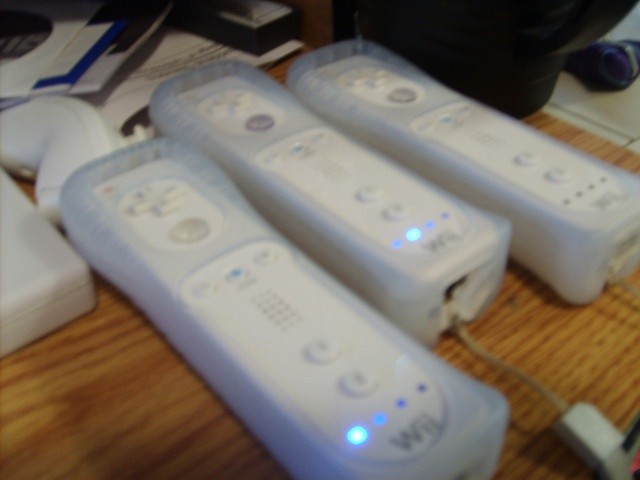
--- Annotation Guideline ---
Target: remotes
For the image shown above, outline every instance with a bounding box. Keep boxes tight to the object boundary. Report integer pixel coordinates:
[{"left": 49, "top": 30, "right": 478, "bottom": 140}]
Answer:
[
  {"left": 290, "top": 36, "right": 635, "bottom": 308},
  {"left": 149, "top": 60, "right": 511, "bottom": 348},
  {"left": 61, "top": 137, "right": 509, "bottom": 480}
]
